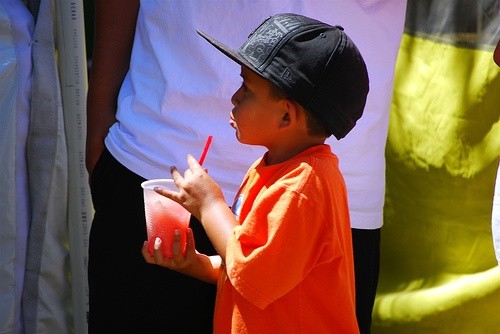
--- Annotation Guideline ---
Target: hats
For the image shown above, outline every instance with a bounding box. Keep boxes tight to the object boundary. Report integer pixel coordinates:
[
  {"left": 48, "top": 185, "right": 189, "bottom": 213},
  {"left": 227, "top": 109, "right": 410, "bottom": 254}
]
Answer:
[{"left": 195, "top": 14, "right": 371, "bottom": 141}]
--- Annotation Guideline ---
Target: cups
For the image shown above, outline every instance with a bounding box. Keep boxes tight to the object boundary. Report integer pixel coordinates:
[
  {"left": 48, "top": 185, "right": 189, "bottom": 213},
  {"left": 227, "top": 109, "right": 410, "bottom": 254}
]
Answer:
[{"left": 141, "top": 179, "right": 192, "bottom": 258}]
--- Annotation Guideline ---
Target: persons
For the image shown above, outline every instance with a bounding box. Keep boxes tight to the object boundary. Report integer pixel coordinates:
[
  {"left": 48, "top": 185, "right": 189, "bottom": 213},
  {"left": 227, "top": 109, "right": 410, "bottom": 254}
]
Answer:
[
  {"left": 141, "top": 12, "right": 370, "bottom": 334},
  {"left": 85, "top": 0, "right": 409, "bottom": 334}
]
